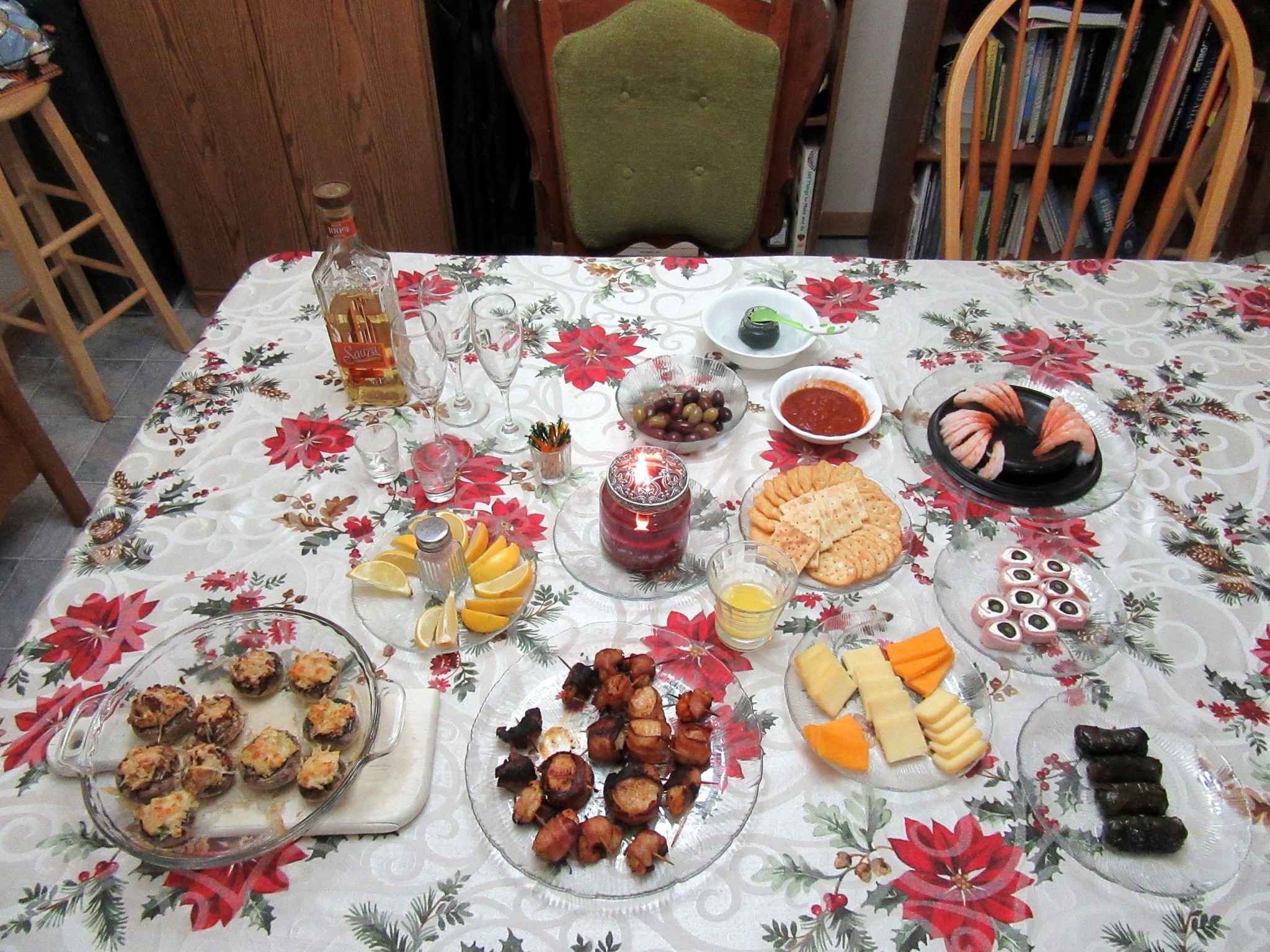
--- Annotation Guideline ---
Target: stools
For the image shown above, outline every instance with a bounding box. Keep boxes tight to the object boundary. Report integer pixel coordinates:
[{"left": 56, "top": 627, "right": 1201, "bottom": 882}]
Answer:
[{"left": 0, "top": 63, "right": 195, "bottom": 421}]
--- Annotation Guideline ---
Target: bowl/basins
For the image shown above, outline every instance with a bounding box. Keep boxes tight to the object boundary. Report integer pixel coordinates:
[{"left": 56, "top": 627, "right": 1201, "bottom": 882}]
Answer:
[
  {"left": 614, "top": 355, "right": 748, "bottom": 453},
  {"left": 769, "top": 364, "right": 881, "bottom": 445},
  {"left": 59, "top": 605, "right": 408, "bottom": 870},
  {"left": 701, "top": 287, "right": 821, "bottom": 371}
]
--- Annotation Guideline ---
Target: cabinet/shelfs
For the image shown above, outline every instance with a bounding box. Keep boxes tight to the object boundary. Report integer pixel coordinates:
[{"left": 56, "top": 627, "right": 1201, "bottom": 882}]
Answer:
[{"left": 866, "top": 0, "right": 1270, "bottom": 262}]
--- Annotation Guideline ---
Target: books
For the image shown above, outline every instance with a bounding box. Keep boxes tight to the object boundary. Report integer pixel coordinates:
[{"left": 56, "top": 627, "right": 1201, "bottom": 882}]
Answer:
[
  {"left": 787, "top": 137, "right": 819, "bottom": 257},
  {"left": 906, "top": 0, "right": 1233, "bottom": 264}
]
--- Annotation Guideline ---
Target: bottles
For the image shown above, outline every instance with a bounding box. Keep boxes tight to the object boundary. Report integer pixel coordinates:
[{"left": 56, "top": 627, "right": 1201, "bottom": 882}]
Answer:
[
  {"left": 309, "top": 179, "right": 413, "bottom": 408},
  {"left": 411, "top": 518, "right": 468, "bottom": 600}
]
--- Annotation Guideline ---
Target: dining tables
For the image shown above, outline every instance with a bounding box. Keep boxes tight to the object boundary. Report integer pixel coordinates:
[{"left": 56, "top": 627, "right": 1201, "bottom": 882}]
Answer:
[{"left": 0, "top": 248, "right": 1270, "bottom": 952}]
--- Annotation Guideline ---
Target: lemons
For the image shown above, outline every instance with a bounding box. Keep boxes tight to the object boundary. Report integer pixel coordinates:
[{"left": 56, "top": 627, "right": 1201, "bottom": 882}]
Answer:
[{"left": 347, "top": 511, "right": 533, "bottom": 650}]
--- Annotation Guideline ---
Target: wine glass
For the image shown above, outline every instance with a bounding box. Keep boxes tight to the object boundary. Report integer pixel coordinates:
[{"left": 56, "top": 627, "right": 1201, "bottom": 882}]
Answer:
[
  {"left": 417, "top": 272, "right": 490, "bottom": 427},
  {"left": 389, "top": 309, "right": 472, "bottom": 470},
  {"left": 468, "top": 291, "right": 534, "bottom": 453}
]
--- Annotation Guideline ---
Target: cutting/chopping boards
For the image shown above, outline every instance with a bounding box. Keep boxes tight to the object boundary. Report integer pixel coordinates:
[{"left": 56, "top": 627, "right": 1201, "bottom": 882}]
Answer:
[{"left": 42, "top": 664, "right": 440, "bottom": 840}]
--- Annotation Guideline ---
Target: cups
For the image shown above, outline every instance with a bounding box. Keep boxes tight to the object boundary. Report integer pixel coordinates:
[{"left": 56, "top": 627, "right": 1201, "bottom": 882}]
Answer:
[
  {"left": 353, "top": 424, "right": 402, "bottom": 483},
  {"left": 705, "top": 540, "right": 800, "bottom": 651},
  {"left": 528, "top": 427, "right": 571, "bottom": 485},
  {"left": 597, "top": 448, "right": 693, "bottom": 571},
  {"left": 411, "top": 444, "right": 455, "bottom": 502}
]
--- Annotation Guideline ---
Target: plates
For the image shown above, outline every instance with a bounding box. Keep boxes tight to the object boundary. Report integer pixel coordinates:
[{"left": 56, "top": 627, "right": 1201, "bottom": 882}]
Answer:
[
  {"left": 730, "top": 466, "right": 915, "bottom": 594},
  {"left": 933, "top": 525, "right": 1125, "bottom": 680},
  {"left": 461, "top": 616, "right": 767, "bottom": 900},
  {"left": 899, "top": 360, "right": 1141, "bottom": 516},
  {"left": 1013, "top": 688, "right": 1252, "bottom": 897},
  {"left": 550, "top": 470, "right": 731, "bottom": 603},
  {"left": 783, "top": 609, "right": 994, "bottom": 792},
  {"left": 351, "top": 504, "right": 540, "bottom": 655}
]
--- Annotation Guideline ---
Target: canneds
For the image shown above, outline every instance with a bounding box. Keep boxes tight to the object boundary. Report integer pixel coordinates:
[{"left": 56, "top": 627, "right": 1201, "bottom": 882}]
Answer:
[{"left": 597, "top": 447, "right": 690, "bottom": 573}]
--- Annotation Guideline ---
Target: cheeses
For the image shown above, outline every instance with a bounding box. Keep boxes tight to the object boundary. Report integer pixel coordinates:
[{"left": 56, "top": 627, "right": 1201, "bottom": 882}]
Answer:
[{"left": 793, "top": 625, "right": 988, "bottom": 776}]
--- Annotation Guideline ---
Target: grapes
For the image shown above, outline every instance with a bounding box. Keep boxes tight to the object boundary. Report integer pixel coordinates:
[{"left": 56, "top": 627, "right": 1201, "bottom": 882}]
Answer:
[{"left": 635, "top": 389, "right": 732, "bottom": 443}]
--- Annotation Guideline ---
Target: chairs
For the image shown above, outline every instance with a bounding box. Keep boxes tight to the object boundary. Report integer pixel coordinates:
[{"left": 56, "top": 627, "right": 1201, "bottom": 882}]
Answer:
[
  {"left": 941, "top": 0, "right": 1252, "bottom": 262},
  {"left": 494, "top": 0, "right": 837, "bottom": 256}
]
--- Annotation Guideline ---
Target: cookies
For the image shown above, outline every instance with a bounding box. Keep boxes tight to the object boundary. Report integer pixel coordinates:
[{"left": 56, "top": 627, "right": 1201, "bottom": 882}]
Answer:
[{"left": 747, "top": 459, "right": 902, "bottom": 586}]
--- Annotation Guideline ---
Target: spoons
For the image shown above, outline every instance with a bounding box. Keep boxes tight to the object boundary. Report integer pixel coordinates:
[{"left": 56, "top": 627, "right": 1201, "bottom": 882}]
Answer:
[{"left": 751, "top": 310, "right": 848, "bottom": 337}]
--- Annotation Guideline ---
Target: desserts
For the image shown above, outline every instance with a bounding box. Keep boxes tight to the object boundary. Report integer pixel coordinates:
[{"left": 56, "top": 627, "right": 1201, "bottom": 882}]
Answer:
[
  {"left": 971, "top": 546, "right": 1090, "bottom": 652},
  {"left": 1073, "top": 724, "right": 1187, "bottom": 855},
  {"left": 115, "top": 649, "right": 359, "bottom": 846}
]
